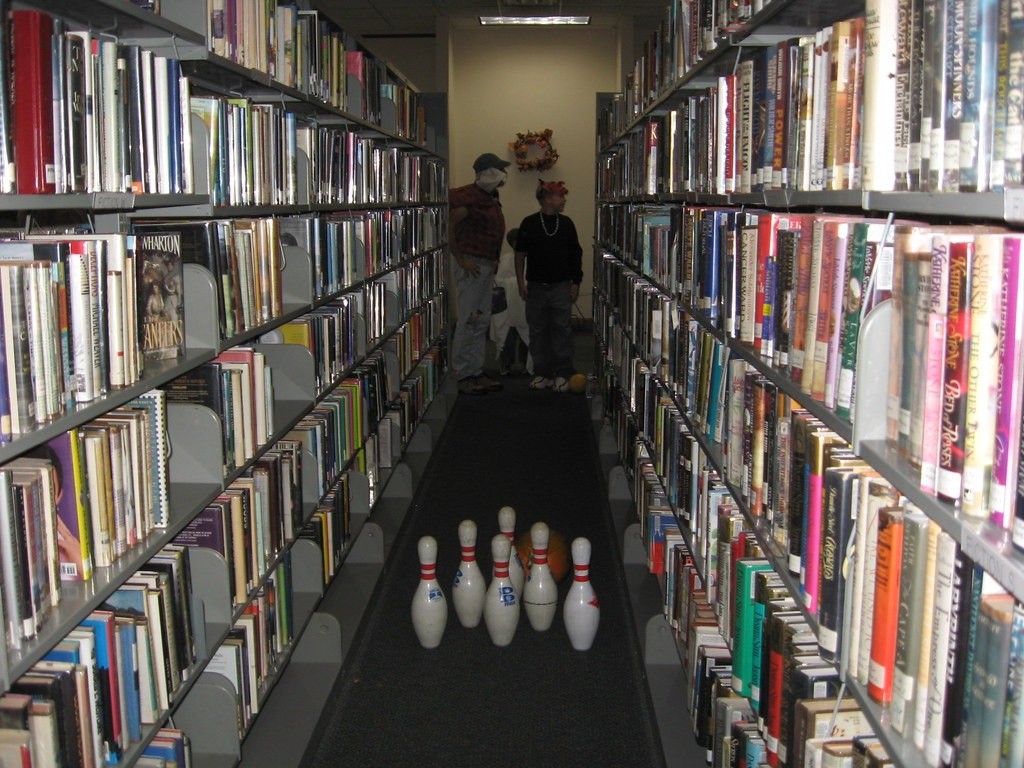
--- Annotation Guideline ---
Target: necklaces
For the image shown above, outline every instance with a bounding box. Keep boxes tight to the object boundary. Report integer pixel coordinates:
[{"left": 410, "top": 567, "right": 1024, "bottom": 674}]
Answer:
[{"left": 540, "top": 212, "right": 559, "bottom": 236}]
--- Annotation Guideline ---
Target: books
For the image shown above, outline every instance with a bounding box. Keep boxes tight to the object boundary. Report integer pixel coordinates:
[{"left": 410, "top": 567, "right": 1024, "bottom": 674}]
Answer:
[
  {"left": 0, "top": 0, "right": 447, "bottom": 768},
  {"left": 590, "top": 0, "right": 1024, "bottom": 768}
]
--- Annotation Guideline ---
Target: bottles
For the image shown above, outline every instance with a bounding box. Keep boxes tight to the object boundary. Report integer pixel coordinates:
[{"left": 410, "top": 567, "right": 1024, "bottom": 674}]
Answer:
[{"left": 586, "top": 373, "right": 594, "bottom": 399}]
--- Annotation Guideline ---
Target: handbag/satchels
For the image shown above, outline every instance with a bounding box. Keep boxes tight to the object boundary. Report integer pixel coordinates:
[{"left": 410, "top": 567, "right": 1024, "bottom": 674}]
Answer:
[{"left": 491, "top": 280, "right": 507, "bottom": 315}]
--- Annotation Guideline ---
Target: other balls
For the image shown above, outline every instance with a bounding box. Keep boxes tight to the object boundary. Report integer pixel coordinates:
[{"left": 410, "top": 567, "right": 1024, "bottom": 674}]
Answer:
[
  {"left": 570, "top": 374, "right": 586, "bottom": 393},
  {"left": 515, "top": 528, "right": 570, "bottom": 583}
]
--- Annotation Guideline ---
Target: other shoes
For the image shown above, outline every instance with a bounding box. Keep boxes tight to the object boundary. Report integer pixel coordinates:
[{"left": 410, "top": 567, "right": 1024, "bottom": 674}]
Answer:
[
  {"left": 486, "top": 376, "right": 504, "bottom": 391},
  {"left": 457, "top": 372, "right": 489, "bottom": 395}
]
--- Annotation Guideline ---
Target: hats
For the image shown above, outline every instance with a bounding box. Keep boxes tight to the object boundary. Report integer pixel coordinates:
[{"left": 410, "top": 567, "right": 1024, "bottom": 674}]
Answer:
[
  {"left": 472, "top": 152, "right": 511, "bottom": 173},
  {"left": 536, "top": 179, "right": 568, "bottom": 200}
]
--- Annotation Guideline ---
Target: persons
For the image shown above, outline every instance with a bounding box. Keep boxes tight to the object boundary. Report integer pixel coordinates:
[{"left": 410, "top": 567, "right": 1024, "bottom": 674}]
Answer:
[
  {"left": 449, "top": 153, "right": 511, "bottom": 395},
  {"left": 515, "top": 179, "right": 584, "bottom": 391},
  {"left": 489, "top": 228, "right": 529, "bottom": 375}
]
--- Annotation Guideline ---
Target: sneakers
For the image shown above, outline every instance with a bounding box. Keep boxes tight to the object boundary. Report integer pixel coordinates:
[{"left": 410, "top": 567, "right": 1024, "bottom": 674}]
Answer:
[
  {"left": 552, "top": 376, "right": 570, "bottom": 393},
  {"left": 528, "top": 376, "right": 553, "bottom": 390}
]
